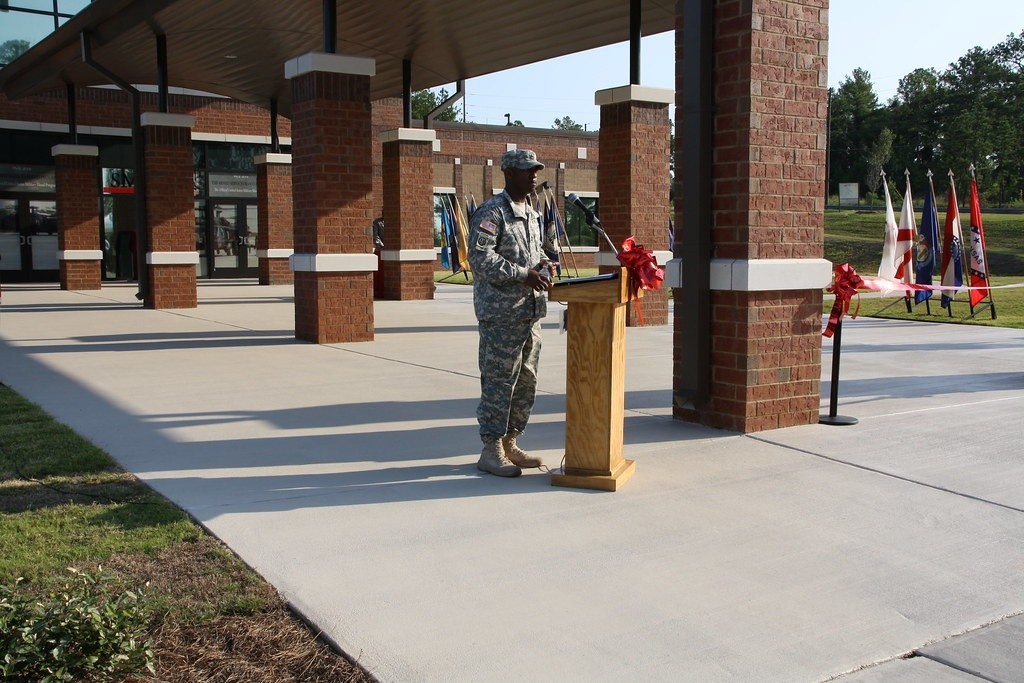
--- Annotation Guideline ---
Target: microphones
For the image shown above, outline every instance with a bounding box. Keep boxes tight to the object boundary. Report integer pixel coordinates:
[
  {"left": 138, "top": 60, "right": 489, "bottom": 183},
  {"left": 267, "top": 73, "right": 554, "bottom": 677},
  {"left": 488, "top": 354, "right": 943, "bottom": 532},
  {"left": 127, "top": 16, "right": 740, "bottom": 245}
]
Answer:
[{"left": 568, "top": 192, "right": 600, "bottom": 224}]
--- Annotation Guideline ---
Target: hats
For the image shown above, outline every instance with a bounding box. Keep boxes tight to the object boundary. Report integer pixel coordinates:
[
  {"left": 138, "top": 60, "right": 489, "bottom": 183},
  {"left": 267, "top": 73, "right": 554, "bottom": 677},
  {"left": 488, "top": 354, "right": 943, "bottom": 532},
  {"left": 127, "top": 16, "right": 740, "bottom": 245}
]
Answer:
[{"left": 501, "top": 149, "right": 545, "bottom": 171}]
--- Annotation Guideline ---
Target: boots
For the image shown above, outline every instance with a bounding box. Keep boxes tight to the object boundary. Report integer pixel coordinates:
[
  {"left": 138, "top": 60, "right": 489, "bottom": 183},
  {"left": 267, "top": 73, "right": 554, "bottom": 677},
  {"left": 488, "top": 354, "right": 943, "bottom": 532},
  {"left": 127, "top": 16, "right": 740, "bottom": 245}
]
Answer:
[
  {"left": 476, "top": 438, "right": 521, "bottom": 477},
  {"left": 502, "top": 434, "right": 542, "bottom": 468}
]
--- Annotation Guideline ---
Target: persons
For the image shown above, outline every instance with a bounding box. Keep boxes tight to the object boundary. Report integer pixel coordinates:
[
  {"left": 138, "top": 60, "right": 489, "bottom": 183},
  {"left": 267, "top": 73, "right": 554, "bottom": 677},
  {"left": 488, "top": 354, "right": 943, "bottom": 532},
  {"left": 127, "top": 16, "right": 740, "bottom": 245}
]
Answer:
[
  {"left": 373, "top": 205, "right": 384, "bottom": 251},
  {"left": 466, "top": 149, "right": 560, "bottom": 477}
]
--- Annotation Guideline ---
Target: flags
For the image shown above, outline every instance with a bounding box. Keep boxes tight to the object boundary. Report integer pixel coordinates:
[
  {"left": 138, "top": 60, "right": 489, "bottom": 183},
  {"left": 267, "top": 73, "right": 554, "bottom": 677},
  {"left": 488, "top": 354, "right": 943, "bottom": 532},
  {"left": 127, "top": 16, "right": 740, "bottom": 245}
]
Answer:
[
  {"left": 876, "top": 183, "right": 904, "bottom": 298},
  {"left": 441, "top": 199, "right": 477, "bottom": 273},
  {"left": 915, "top": 188, "right": 939, "bottom": 305},
  {"left": 893, "top": 188, "right": 916, "bottom": 301},
  {"left": 940, "top": 188, "right": 964, "bottom": 309},
  {"left": 968, "top": 180, "right": 989, "bottom": 307},
  {"left": 535, "top": 197, "right": 565, "bottom": 281}
]
